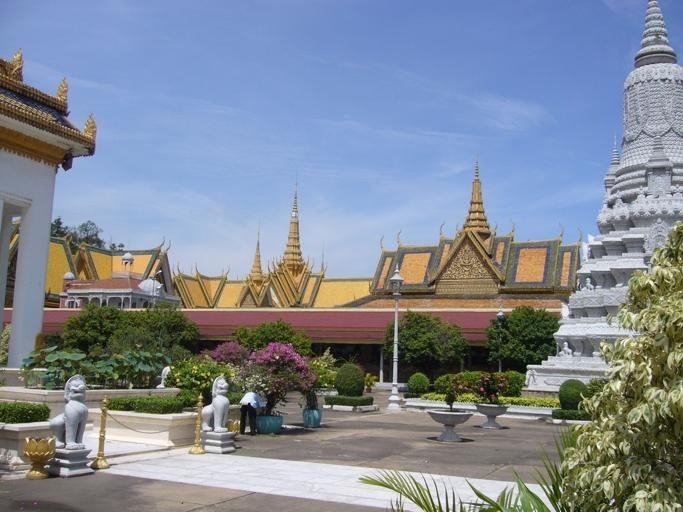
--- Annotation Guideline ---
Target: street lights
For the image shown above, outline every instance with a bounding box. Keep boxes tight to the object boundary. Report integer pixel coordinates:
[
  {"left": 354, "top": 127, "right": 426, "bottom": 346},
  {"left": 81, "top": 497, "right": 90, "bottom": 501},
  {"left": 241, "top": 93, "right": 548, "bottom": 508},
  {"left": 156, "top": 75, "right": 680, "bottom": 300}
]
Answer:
[
  {"left": 150, "top": 269, "right": 164, "bottom": 306},
  {"left": 387, "top": 262, "right": 406, "bottom": 412},
  {"left": 497, "top": 301, "right": 504, "bottom": 374}
]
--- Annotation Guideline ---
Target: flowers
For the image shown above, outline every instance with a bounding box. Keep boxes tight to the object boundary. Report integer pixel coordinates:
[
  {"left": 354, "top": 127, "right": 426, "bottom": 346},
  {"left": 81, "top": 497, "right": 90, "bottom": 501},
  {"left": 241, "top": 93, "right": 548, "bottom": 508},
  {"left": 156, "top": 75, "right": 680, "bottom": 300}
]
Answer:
[
  {"left": 231, "top": 342, "right": 315, "bottom": 416},
  {"left": 298, "top": 347, "right": 338, "bottom": 410}
]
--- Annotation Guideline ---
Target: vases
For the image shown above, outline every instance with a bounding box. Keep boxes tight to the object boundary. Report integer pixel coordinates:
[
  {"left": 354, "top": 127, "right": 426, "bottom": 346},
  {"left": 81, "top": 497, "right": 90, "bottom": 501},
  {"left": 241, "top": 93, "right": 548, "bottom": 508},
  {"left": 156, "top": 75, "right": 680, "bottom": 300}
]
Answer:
[
  {"left": 256, "top": 416, "right": 283, "bottom": 434},
  {"left": 303, "top": 410, "right": 321, "bottom": 427}
]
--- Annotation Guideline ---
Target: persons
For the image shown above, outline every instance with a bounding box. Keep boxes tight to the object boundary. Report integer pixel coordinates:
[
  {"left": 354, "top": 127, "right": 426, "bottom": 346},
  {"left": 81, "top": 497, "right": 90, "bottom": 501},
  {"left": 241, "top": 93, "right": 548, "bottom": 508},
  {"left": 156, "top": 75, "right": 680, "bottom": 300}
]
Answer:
[{"left": 237, "top": 391, "right": 261, "bottom": 436}]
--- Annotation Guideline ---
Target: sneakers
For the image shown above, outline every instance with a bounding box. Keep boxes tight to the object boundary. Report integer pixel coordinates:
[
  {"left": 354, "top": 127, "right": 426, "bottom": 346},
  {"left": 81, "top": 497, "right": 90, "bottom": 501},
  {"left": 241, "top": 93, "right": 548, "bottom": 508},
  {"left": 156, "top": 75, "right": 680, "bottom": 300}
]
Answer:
[
  {"left": 251, "top": 430, "right": 256, "bottom": 436},
  {"left": 240, "top": 430, "right": 245, "bottom": 435}
]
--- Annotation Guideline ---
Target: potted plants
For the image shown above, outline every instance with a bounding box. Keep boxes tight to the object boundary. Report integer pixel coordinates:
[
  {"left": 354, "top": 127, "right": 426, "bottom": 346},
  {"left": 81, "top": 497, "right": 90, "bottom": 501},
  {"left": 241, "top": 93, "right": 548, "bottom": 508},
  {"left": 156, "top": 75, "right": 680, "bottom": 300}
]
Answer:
[{"left": 364, "top": 373, "right": 377, "bottom": 392}]
